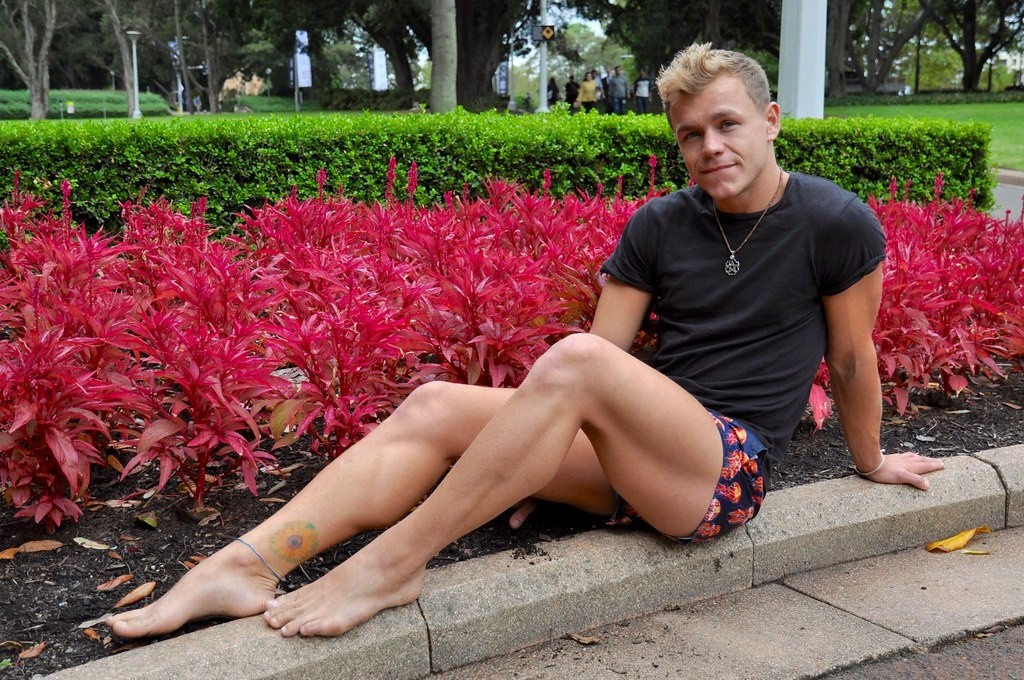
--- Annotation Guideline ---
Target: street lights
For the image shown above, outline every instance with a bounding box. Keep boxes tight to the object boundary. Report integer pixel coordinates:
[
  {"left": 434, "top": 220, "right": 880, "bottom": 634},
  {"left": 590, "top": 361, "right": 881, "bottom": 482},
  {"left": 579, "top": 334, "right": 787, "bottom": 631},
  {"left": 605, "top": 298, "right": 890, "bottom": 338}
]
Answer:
[{"left": 126, "top": 31, "right": 144, "bottom": 120}]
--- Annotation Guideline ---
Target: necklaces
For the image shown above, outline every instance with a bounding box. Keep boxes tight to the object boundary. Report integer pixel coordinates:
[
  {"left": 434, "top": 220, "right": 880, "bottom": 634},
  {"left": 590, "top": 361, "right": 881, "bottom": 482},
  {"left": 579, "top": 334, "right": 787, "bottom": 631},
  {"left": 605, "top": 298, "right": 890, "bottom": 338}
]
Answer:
[{"left": 713, "top": 166, "right": 783, "bottom": 276}]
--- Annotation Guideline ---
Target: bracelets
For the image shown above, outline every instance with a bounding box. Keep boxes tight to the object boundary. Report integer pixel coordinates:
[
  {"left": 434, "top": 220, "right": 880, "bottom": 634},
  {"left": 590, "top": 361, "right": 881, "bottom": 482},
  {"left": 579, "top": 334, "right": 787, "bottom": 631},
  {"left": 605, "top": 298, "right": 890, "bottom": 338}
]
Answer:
[{"left": 855, "top": 449, "right": 886, "bottom": 476}]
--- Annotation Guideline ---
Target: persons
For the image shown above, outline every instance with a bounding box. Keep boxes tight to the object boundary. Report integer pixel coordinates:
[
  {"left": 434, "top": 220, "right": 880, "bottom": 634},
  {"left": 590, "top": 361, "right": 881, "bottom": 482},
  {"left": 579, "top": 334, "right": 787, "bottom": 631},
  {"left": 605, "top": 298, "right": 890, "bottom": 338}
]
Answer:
[
  {"left": 107, "top": 43, "right": 944, "bottom": 637},
  {"left": 608, "top": 66, "right": 630, "bottom": 116},
  {"left": 634, "top": 67, "right": 651, "bottom": 115},
  {"left": 565, "top": 76, "right": 580, "bottom": 114},
  {"left": 576, "top": 72, "right": 597, "bottom": 114},
  {"left": 592, "top": 70, "right": 605, "bottom": 113},
  {"left": 547, "top": 77, "right": 559, "bottom": 107},
  {"left": 601, "top": 68, "right": 613, "bottom": 114},
  {"left": 525, "top": 92, "right": 531, "bottom": 104}
]
